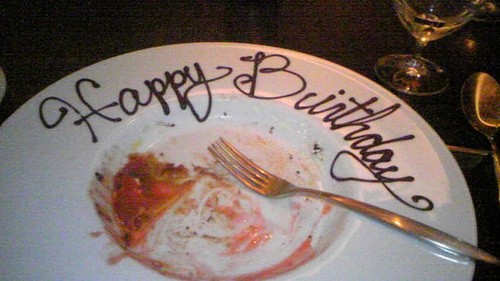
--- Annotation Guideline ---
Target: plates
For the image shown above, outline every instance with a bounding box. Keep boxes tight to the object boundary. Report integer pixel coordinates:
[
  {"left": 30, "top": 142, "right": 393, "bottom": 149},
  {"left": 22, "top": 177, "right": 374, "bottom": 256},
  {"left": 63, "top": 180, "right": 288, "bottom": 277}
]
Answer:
[{"left": 0, "top": 42, "right": 500, "bottom": 281}]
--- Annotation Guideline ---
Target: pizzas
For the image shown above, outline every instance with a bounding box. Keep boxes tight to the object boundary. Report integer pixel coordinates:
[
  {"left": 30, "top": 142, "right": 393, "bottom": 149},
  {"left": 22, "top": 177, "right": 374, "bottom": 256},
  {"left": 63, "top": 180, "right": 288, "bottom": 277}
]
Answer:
[{"left": 111, "top": 153, "right": 198, "bottom": 248}]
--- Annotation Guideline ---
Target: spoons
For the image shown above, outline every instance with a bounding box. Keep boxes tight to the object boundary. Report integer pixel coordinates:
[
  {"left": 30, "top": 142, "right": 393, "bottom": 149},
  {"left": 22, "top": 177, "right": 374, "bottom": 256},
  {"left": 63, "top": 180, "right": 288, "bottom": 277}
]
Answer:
[{"left": 461, "top": 72, "right": 500, "bottom": 203}]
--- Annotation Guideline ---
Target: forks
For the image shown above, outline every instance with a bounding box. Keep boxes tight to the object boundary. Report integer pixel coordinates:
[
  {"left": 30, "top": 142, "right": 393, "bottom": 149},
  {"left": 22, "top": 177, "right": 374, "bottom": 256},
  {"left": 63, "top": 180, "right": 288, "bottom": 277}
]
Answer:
[{"left": 208, "top": 136, "right": 500, "bottom": 272}]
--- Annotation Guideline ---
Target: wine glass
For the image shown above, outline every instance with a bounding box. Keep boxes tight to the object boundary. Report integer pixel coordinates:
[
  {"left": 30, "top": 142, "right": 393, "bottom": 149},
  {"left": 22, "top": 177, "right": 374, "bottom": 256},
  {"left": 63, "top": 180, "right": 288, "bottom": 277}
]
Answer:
[{"left": 372, "top": 1, "right": 482, "bottom": 98}]
